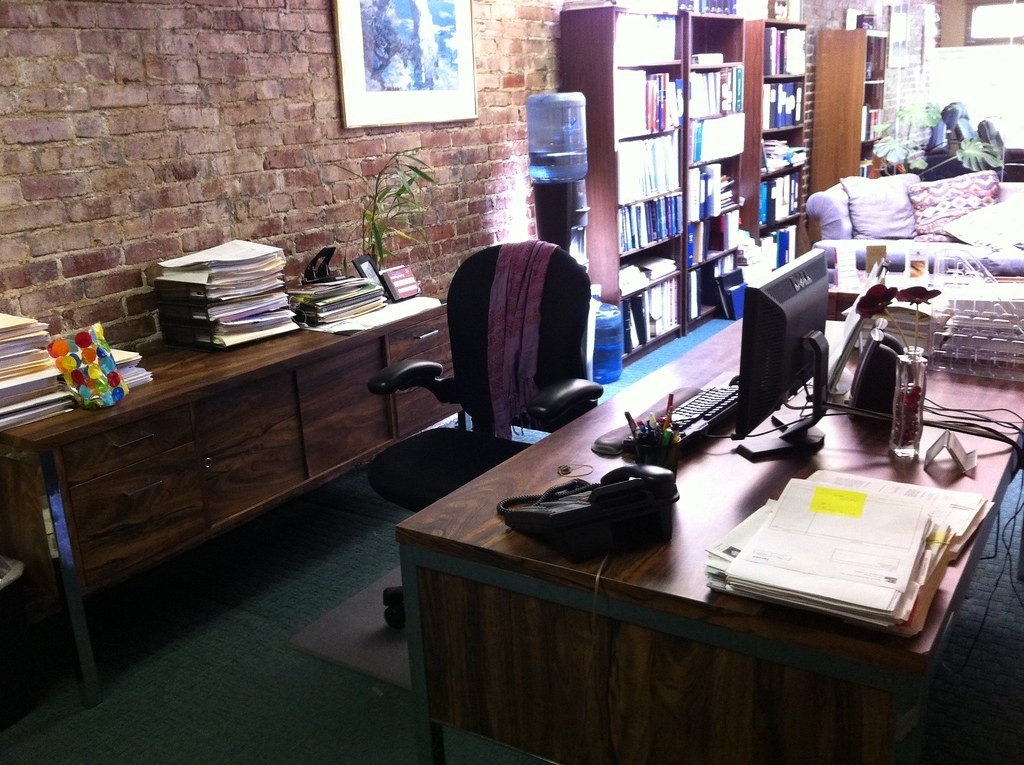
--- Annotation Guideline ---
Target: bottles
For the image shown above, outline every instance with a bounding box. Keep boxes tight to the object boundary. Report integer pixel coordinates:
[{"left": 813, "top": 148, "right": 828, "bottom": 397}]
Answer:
[
  {"left": 589, "top": 283, "right": 623, "bottom": 384},
  {"left": 526, "top": 91, "right": 588, "bottom": 181}
]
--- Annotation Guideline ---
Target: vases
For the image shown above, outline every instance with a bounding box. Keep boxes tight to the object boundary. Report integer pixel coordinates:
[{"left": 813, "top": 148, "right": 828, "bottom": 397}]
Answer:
[{"left": 888, "top": 345, "right": 929, "bottom": 459}]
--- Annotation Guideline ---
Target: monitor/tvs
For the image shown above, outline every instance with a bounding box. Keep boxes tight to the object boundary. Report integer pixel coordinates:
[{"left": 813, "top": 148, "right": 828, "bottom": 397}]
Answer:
[{"left": 734, "top": 249, "right": 830, "bottom": 462}]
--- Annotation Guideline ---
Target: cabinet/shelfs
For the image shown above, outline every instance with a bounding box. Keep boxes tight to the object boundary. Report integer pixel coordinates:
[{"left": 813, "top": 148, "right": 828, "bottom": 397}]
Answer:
[
  {"left": 807, "top": 27, "right": 891, "bottom": 246},
  {"left": 0, "top": 295, "right": 464, "bottom": 709},
  {"left": 559, "top": 6, "right": 805, "bottom": 371}
]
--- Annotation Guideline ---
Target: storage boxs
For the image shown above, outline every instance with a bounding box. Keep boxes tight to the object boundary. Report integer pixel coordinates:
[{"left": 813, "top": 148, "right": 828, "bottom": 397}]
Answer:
[
  {"left": 692, "top": 54, "right": 724, "bottom": 64},
  {"left": 759, "top": 184, "right": 768, "bottom": 224},
  {"left": 929, "top": 247, "right": 1024, "bottom": 383},
  {"left": 857, "top": 14, "right": 877, "bottom": 30},
  {"left": 768, "top": 181, "right": 776, "bottom": 222},
  {"left": 728, "top": 282, "right": 748, "bottom": 321},
  {"left": 794, "top": 173, "right": 799, "bottom": 214},
  {"left": 767, "top": 0, "right": 790, "bottom": 20},
  {"left": 719, "top": 269, "right": 744, "bottom": 319},
  {"left": 789, "top": 174, "right": 795, "bottom": 215}
]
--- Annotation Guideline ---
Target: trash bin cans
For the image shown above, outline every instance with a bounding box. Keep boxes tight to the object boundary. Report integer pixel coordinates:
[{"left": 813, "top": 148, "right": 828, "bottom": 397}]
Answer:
[{"left": 0, "top": 556, "right": 43, "bottom": 732}]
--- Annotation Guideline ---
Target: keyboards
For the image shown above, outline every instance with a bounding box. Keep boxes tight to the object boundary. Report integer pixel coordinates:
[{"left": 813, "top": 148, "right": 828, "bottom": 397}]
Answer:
[{"left": 622, "top": 382, "right": 739, "bottom": 458}]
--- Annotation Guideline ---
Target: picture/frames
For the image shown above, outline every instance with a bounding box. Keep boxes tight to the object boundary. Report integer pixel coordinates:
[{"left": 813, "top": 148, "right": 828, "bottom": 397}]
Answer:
[
  {"left": 905, "top": 254, "right": 929, "bottom": 286},
  {"left": 333, "top": 0, "right": 480, "bottom": 129},
  {"left": 351, "top": 254, "right": 391, "bottom": 299},
  {"left": 886, "top": 11, "right": 909, "bottom": 70}
]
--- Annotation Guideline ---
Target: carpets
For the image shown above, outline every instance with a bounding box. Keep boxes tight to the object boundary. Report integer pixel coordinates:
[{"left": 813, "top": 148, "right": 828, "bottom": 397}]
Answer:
[{"left": 286, "top": 563, "right": 412, "bottom": 690}]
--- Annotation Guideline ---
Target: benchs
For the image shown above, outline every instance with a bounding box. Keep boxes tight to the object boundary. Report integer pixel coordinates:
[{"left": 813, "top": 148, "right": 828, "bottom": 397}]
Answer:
[{"left": 805, "top": 181, "right": 1024, "bottom": 288}]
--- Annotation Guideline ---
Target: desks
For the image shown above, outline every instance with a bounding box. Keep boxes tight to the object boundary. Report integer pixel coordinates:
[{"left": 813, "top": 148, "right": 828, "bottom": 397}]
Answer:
[{"left": 392, "top": 314, "right": 1024, "bottom": 765}]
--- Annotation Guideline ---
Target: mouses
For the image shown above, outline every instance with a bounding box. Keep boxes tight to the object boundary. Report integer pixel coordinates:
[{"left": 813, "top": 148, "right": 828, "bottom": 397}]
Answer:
[{"left": 730, "top": 375, "right": 741, "bottom": 386}]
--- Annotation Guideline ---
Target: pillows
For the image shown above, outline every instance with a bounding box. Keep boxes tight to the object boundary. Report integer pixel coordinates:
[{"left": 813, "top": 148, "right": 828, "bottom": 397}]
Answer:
[
  {"left": 943, "top": 190, "right": 1024, "bottom": 248},
  {"left": 906, "top": 170, "right": 1000, "bottom": 244},
  {"left": 839, "top": 172, "right": 921, "bottom": 240}
]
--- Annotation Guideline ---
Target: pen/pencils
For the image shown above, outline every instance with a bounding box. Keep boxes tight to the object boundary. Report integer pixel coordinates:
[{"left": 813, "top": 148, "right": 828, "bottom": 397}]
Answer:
[
  {"left": 638, "top": 412, "right": 681, "bottom": 465},
  {"left": 625, "top": 412, "right": 637, "bottom": 438},
  {"left": 666, "top": 394, "right": 673, "bottom": 415},
  {"left": 662, "top": 406, "right": 673, "bottom": 430}
]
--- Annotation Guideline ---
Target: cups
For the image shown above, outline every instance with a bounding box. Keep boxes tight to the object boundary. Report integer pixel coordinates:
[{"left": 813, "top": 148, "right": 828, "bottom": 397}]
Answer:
[{"left": 636, "top": 441, "right": 679, "bottom": 501}]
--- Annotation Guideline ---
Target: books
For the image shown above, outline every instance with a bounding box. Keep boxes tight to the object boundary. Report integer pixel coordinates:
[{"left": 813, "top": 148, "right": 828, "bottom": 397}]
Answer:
[
  {"left": 757, "top": 176, "right": 801, "bottom": 226},
  {"left": 689, "top": 112, "right": 747, "bottom": 167},
  {"left": 688, "top": 67, "right": 747, "bottom": 117},
  {"left": 616, "top": 193, "right": 685, "bottom": 254},
  {"left": 861, "top": 104, "right": 884, "bottom": 142},
  {"left": 618, "top": 259, "right": 677, "bottom": 295},
  {"left": 684, "top": 165, "right": 744, "bottom": 222},
  {"left": 694, "top": 53, "right": 725, "bottom": 66},
  {"left": 622, "top": 281, "right": 681, "bottom": 349},
  {"left": 742, "top": 224, "right": 798, "bottom": 283},
  {"left": 1, "top": 312, "right": 154, "bottom": 431},
  {"left": 686, "top": 213, "right": 749, "bottom": 320},
  {"left": 762, "top": 84, "right": 803, "bottom": 130},
  {"left": 616, "top": 135, "right": 682, "bottom": 202},
  {"left": 767, "top": 27, "right": 806, "bottom": 77},
  {"left": 615, "top": 70, "right": 686, "bottom": 139},
  {"left": 614, "top": 16, "right": 677, "bottom": 66},
  {"left": 761, "top": 138, "right": 808, "bottom": 174},
  {"left": 697, "top": 0, "right": 738, "bottom": 17}
]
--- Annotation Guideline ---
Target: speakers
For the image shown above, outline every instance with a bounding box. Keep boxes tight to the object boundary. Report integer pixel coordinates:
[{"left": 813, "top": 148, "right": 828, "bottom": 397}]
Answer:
[{"left": 848, "top": 320, "right": 905, "bottom": 418}]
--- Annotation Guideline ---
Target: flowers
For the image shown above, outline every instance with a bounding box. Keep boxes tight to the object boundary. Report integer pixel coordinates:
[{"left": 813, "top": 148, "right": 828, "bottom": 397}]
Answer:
[{"left": 854, "top": 283, "right": 942, "bottom": 386}]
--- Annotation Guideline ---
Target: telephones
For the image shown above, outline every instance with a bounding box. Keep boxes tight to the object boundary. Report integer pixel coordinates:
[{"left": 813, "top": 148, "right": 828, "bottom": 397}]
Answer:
[{"left": 505, "top": 465, "right": 679, "bottom": 563}]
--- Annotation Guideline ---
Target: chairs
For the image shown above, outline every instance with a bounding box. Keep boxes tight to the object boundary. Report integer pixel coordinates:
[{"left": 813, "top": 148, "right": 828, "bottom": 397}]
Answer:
[
  {"left": 365, "top": 240, "right": 603, "bottom": 631},
  {"left": 919, "top": 101, "right": 1024, "bottom": 182}
]
